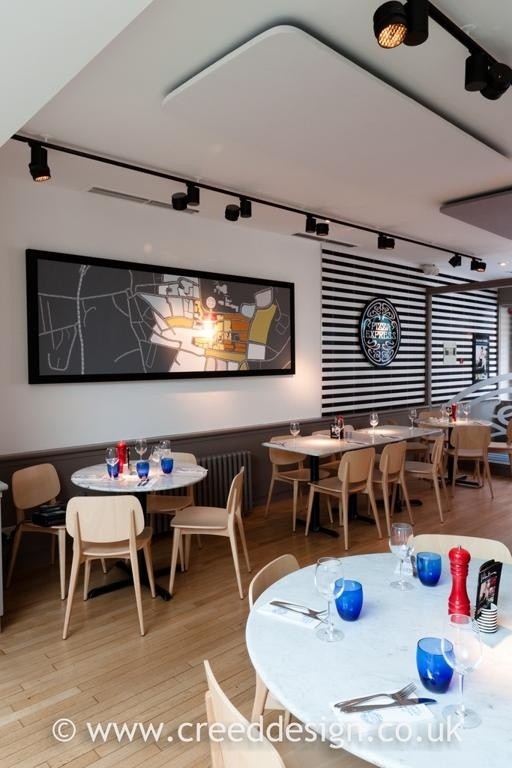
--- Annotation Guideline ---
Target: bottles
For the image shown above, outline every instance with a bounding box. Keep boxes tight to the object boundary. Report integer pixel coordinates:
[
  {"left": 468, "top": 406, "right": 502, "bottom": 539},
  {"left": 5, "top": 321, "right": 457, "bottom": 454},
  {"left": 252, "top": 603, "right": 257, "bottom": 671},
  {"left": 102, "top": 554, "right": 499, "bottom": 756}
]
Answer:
[
  {"left": 448, "top": 543, "right": 473, "bottom": 625},
  {"left": 116, "top": 440, "right": 127, "bottom": 472}
]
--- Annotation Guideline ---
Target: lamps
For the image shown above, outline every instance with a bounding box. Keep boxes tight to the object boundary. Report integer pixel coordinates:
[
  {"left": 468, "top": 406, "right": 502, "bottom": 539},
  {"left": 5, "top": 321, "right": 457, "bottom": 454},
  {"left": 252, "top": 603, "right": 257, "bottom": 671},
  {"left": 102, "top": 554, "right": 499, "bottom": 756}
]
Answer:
[
  {"left": 11, "top": 135, "right": 486, "bottom": 273},
  {"left": 371, "top": 0, "right": 512, "bottom": 101}
]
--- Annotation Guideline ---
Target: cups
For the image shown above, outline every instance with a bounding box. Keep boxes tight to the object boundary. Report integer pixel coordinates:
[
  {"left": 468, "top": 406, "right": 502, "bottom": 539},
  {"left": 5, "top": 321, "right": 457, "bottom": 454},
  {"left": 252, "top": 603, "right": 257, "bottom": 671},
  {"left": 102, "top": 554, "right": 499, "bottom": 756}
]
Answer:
[
  {"left": 151, "top": 438, "right": 181, "bottom": 463},
  {"left": 160, "top": 457, "right": 174, "bottom": 473},
  {"left": 416, "top": 552, "right": 442, "bottom": 587},
  {"left": 417, "top": 634, "right": 454, "bottom": 698},
  {"left": 106, "top": 463, "right": 119, "bottom": 479},
  {"left": 136, "top": 461, "right": 152, "bottom": 479},
  {"left": 335, "top": 578, "right": 363, "bottom": 622}
]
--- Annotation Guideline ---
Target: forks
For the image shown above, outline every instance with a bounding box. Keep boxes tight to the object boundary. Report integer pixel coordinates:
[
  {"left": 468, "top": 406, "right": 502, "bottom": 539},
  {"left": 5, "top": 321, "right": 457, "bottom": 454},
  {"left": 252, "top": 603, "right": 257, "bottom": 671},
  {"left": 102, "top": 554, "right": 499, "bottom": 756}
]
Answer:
[
  {"left": 137, "top": 479, "right": 145, "bottom": 485},
  {"left": 333, "top": 679, "right": 417, "bottom": 710},
  {"left": 272, "top": 600, "right": 328, "bottom": 616}
]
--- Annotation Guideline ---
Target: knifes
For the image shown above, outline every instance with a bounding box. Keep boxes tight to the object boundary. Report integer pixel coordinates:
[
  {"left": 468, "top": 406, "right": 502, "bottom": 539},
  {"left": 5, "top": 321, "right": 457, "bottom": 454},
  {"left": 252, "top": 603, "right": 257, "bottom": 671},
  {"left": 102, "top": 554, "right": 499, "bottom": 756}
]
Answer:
[
  {"left": 141, "top": 478, "right": 150, "bottom": 485},
  {"left": 273, "top": 603, "right": 326, "bottom": 629},
  {"left": 336, "top": 697, "right": 439, "bottom": 708}
]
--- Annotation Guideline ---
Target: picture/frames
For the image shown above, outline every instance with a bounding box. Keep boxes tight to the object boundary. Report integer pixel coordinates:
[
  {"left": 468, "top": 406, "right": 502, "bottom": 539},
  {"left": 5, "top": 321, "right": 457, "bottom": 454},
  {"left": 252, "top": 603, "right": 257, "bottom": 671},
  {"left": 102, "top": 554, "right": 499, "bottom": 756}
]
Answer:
[{"left": 28, "top": 248, "right": 296, "bottom": 384}]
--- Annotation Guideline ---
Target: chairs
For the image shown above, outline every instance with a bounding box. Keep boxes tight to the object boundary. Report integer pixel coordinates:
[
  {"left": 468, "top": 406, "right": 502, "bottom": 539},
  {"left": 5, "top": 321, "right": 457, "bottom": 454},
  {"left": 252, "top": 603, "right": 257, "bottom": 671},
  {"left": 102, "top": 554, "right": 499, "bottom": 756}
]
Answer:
[
  {"left": 1, "top": 408, "right": 512, "bottom": 641},
  {"left": 201, "top": 658, "right": 378, "bottom": 768},
  {"left": 249, "top": 553, "right": 327, "bottom": 738}
]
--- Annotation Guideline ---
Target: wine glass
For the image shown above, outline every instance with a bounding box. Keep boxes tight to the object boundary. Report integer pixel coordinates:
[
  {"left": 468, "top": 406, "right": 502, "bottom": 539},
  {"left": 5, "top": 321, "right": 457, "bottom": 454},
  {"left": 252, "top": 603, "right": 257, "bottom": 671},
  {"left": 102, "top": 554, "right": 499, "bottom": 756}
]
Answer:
[
  {"left": 437, "top": 607, "right": 485, "bottom": 733},
  {"left": 287, "top": 404, "right": 419, "bottom": 444},
  {"left": 105, "top": 445, "right": 123, "bottom": 480},
  {"left": 438, "top": 400, "right": 473, "bottom": 422},
  {"left": 134, "top": 436, "right": 149, "bottom": 461},
  {"left": 313, "top": 552, "right": 345, "bottom": 644},
  {"left": 387, "top": 520, "right": 416, "bottom": 593}
]
